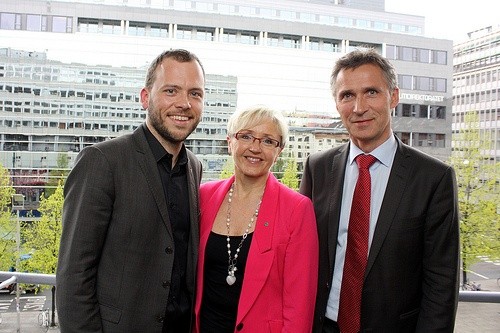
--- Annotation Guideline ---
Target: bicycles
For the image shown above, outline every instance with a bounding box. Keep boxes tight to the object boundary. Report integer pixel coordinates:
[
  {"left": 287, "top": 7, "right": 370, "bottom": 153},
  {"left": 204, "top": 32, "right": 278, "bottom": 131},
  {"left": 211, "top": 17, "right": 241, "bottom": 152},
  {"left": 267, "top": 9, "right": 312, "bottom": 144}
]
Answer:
[{"left": 38, "top": 308, "right": 50, "bottom": 333}]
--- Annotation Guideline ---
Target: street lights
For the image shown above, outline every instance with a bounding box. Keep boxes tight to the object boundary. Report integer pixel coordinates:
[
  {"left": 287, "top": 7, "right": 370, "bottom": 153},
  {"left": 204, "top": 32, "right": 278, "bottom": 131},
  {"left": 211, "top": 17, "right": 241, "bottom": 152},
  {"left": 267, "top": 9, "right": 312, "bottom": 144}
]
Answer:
[{"left": 10, "top": 192, "right": 26, "bottom": 333}]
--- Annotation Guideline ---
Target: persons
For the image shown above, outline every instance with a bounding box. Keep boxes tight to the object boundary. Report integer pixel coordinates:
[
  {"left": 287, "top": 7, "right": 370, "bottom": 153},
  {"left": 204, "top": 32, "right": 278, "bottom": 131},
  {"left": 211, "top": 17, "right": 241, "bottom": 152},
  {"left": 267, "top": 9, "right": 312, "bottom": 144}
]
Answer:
[
  {"left": 56, "top": 49, "right": 202, "bottom": 333},
  {"left": 192, "top": 104, "right": 318, "bottom": 333},
  {"left": 299, "top": 48, "right": 460, "bottom": 333}
]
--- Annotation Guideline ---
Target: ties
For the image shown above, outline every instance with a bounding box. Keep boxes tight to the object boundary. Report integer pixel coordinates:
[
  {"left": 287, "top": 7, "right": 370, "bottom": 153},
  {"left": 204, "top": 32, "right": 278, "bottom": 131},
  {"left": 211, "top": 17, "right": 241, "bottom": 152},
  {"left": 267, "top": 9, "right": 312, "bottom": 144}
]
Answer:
[{"left": 337, "top": 154, "right": 376, "bottom": 333}]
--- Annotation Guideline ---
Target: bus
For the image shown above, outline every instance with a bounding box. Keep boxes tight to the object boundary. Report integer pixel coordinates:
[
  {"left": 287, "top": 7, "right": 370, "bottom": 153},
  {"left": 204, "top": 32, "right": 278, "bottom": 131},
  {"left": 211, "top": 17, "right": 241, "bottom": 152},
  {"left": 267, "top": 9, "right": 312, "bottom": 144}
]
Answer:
[{"left": 0, "top": 248, "right": 41, "bottom": 295}]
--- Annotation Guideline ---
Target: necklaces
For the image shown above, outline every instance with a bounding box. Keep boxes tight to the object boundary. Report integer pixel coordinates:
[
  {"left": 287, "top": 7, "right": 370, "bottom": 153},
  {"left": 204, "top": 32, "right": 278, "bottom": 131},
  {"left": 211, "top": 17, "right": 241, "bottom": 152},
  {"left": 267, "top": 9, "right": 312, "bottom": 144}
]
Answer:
[{"left": 226, "top": 183, "right": 266, "bottom": 285}]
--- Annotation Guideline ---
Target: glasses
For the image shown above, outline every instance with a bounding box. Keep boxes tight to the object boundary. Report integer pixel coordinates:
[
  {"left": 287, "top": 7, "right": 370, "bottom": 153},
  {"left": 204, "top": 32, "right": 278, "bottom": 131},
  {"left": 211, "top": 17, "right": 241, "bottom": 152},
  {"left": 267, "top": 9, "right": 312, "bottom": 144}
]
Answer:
[{"left": 232, "top": 132, "right": 281, "bottom": 148}]
